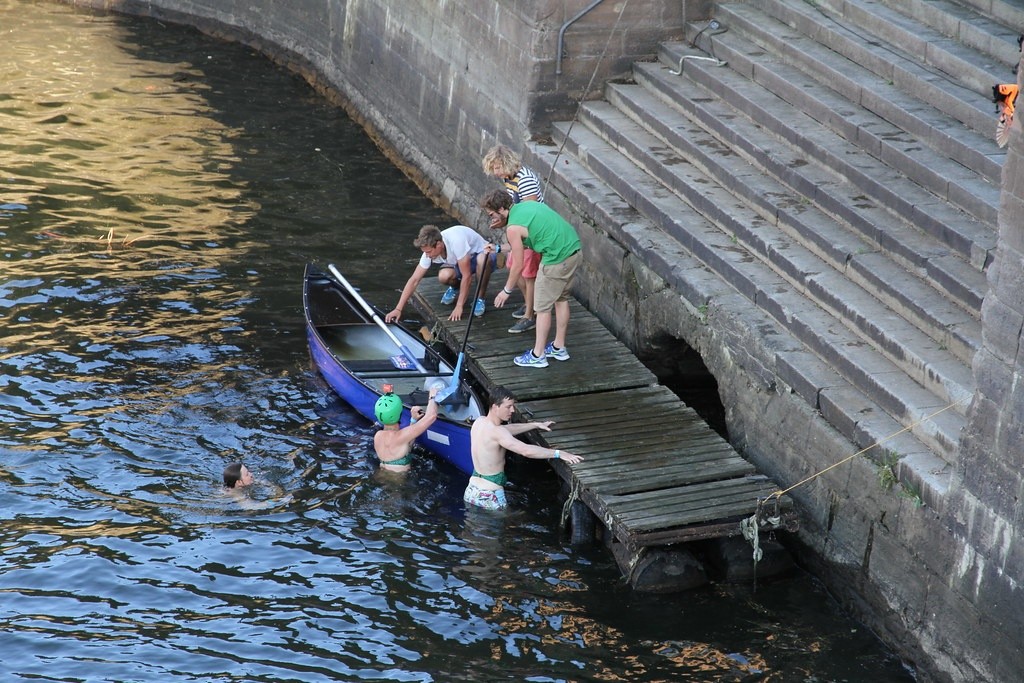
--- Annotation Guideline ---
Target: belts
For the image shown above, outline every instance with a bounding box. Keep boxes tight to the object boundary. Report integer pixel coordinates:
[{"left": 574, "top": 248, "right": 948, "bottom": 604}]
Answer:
[{"left": 570, "top": 249, "right": 581, "bottom": 256}]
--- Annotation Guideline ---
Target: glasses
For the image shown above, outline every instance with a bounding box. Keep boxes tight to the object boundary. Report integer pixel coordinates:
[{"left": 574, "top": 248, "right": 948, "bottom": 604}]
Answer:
[{"left": 488, "top": 211, "right": 495, "bottom": 217}]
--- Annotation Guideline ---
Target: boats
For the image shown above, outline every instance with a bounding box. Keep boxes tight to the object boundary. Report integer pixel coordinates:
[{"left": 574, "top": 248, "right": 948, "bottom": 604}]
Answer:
[{"left": 302, "top": 260, "right": 486, "bottom": 476}]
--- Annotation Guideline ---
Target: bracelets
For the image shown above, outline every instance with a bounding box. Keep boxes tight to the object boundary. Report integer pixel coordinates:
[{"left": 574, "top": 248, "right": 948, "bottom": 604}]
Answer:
[
  {"left": 504, "top": 286, "right": 512, "bottom": 294},
  {"left": 555, "top": 450, "right": 561, "bottom": 458},
  {"left": 496, "top": 244, "right": 501, "bottom": 254},
  {"left": 410, "top": 418, "right": 417, "bottom": 423},
  {"left": 395, "top": 308, "right": 402, "bottom": 312},
  {"left": 427, "top": 396, "right": 435, "bottom": 400}
]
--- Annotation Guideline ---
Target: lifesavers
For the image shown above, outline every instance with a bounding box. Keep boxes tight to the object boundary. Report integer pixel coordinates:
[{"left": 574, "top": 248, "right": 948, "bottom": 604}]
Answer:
[{"left": 560, "top": 496, "right": 596, "bottom": 547}]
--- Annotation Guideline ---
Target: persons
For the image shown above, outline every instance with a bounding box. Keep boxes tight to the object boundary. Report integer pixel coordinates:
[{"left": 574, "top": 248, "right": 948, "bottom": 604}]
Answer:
[
  {"left": 480, "top": 190, "right": 583, "bottom": 367},
  {"left": 464, "top": 386, "right": 585, "bottom": 511},
  {"left": 223, "top": 463, "right": 254, "bottom": 488},
  {"left": 482, "top": 144, "right": 544, "bottom": 333},
  {"left": 374, "top": 388, "right": 438, "bottom": 473},
  {"left": 385, "top": 226, "right": 496, "bottom": 323}
]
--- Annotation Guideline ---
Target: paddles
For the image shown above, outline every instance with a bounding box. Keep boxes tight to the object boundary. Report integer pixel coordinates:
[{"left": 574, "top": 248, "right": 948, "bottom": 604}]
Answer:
[
  {"left": 436, "top": 237, "right": 494, "bottom": 403},
  {"left": 328, "top": 264, "right": 454, "bottom": 411}
]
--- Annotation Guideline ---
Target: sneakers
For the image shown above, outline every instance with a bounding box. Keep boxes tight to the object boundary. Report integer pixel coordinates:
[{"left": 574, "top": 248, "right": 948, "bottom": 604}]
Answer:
[
  {"left": 513, "top": 349, "right": 550, "bottom": 367},
  {"left": 512, "top": 305, "right": 536, "bottom": 317},
  {"left": 544, "top": 340, "right": 570, "bottom": 361},
  {"left": 474, "top": 298, "right": 485, "bottom": 316},
  {"left": 441, "top": 278, "right": 461, "bottom": 305},
  {"left": 509, "top": 317, "right": 536, "bottom": 333}
]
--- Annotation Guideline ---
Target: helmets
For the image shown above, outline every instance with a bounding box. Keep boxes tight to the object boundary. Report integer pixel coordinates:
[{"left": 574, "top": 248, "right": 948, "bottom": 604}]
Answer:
[{"left": 374, "top": 394, "right": 403, "bottom": 425}]
